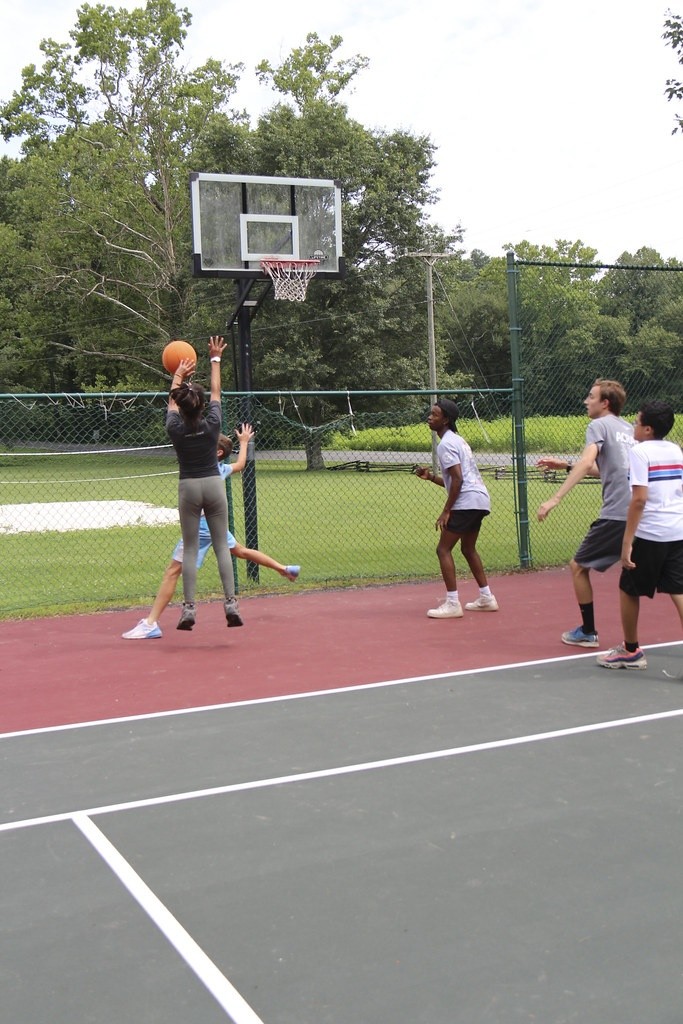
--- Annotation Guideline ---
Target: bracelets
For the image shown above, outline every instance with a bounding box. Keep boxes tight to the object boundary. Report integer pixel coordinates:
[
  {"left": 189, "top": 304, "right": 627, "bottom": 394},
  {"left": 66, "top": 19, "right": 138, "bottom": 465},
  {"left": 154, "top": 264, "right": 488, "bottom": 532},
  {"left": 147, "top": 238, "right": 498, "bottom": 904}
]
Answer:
[
  {"left": 175, "top": 373, "right": 183, "bottom": 378},
  {"left": 431, "top": 475, "right": 436, "bottom": 483},
  {"left": 566, "top": 462, "right": 571, "bottom": 473}
]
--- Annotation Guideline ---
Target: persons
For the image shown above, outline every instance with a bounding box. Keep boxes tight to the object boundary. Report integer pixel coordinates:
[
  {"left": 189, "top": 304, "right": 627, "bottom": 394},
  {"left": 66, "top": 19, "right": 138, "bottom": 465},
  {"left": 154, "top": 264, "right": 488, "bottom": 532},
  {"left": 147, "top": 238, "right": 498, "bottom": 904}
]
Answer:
[
  {"left": 427, "top": 398, "right": 500, "bottom": 619},
  {"left": 119, "top": 423, "right": 302, "bottom": 640},
  {"left": 538, "top": 380, "right": 640, "bottom": 649},
  {"left": 162, "top": 335, "right": 247, "bottom": 632},
  {"left": 596, "top": 403, "right": 683, "bottom": 672}
]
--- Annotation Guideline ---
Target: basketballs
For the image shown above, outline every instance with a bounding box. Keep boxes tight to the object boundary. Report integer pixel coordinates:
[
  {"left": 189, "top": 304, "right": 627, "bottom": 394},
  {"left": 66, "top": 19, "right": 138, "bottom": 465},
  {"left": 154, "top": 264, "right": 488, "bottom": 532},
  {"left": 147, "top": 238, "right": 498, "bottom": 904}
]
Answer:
[{"left": 161, "top": 340, "right": 197, "bottom": 374}]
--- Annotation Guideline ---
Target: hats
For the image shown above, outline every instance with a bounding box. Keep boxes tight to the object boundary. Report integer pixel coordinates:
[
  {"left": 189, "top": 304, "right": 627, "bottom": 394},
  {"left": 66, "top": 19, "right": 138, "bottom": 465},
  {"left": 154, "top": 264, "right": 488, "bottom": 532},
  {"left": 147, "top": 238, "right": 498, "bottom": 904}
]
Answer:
[{"left": 437, "top": 398, "right": 460, "bottom": 433}]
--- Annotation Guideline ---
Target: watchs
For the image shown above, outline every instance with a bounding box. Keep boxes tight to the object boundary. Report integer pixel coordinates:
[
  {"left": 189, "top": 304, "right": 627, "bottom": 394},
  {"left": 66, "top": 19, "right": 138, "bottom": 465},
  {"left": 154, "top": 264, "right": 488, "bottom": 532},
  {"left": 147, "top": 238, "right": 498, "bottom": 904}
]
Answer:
[{"left": 210, "top": 357, "right": 222, "bottom": 362}]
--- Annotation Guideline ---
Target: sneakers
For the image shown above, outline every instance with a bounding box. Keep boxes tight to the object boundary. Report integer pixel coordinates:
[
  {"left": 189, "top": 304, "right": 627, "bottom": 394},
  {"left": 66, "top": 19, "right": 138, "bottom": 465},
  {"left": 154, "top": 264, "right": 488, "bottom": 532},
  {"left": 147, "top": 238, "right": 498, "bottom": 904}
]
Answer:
[
  {"left": 175, "top": 607, "right": 196, "bottom": 631},
  {"left": 427, "top": 600, "right": 464, "bottom": 618},
  {"left": 284, "top": 565, "right": 301, "bottom": 583},
  {"left": 465, "top": 594, "right": 499, "bottom": 611},
  {"left": 224, "top": 602, "right": 243, "bottom": 627},
  {"left": 597, "top": 641, "right": 647, "bottom": 670},
  {"left": 120, "top": 618, "right": 162, "bottom": 638},
  {"left": 561, "top": 627, "right": 600, "bottom": 648}
]
